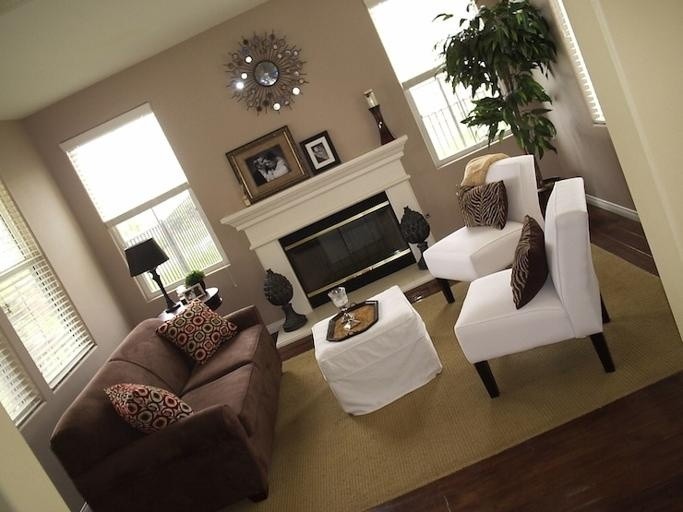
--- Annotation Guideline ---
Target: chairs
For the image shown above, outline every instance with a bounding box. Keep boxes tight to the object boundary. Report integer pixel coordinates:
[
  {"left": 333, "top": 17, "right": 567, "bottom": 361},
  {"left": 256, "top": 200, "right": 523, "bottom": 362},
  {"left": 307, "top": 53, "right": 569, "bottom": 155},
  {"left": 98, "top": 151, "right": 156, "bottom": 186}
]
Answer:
[
  {"left": 454, "top": 177, "right": 616, "bottom": 399},
  {"left": 422, "top": 155, "right": 545, "bottom": 303}
]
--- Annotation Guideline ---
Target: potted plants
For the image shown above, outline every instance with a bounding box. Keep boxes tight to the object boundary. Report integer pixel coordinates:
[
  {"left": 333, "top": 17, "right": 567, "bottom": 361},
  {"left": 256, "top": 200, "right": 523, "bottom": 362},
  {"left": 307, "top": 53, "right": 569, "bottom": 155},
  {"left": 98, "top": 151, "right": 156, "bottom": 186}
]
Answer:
[
  {"left": 184, "top": 269, "right": 207, "bottom": 292},
  {"left": 433, "top": 0, "right": 564, "bottom": 219}
]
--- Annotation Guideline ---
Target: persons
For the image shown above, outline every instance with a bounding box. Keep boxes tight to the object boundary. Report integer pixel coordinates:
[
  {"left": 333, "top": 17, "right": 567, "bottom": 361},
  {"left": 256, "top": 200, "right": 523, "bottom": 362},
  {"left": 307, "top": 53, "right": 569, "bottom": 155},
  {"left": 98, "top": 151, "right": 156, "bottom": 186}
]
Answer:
[
  {"left": 261, "top": 152, "right": 289, "bottom": 182},
  {"left": 252, "top": 155, "right": 267, "bottom": 186},
  {"left": 311, "top": 144, "right": 327, "bottom": 164}
]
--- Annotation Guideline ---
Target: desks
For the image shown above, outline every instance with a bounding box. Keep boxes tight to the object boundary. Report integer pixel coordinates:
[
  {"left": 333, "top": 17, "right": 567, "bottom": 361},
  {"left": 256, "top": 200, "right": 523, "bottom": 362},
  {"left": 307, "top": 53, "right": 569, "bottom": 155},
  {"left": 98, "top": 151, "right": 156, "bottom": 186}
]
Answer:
[{"left": 157, "top": 288, "right": 223, "bottom": 321}]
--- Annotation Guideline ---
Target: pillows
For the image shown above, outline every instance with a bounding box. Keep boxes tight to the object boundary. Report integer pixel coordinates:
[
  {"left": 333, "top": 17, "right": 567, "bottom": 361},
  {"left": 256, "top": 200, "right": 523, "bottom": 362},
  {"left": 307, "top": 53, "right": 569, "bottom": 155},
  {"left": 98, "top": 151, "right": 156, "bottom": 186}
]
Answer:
[
  {"left": 106, "top": 383, "right": 193, "bottom": 433},
  {"left": 510, "top": 214, "right": 549, "bottom": 309},
  {"left": 154, "top": 298, "right": 238, "bottom": 366},
  {"left": 456, "top": 181, "right": 508, "bottom": 230}
]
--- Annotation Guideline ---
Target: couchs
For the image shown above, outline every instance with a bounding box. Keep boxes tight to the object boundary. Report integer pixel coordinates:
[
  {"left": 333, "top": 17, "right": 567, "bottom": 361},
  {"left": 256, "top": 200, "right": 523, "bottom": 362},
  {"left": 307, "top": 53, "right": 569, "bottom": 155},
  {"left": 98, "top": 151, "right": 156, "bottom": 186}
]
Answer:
[{"left": 52, "top": 304, "right": 283, "bottom": 511}]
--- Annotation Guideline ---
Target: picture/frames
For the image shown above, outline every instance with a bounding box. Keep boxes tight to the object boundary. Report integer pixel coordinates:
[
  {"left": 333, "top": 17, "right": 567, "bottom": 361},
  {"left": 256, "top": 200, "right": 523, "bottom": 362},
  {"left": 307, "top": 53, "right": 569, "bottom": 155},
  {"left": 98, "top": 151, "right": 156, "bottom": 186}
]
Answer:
[
  {"left": 226, "top": 126, "right": 310, "bottom": 204},
  {"left": 299, "top": 130, "right": 341, "bottom": 176}
]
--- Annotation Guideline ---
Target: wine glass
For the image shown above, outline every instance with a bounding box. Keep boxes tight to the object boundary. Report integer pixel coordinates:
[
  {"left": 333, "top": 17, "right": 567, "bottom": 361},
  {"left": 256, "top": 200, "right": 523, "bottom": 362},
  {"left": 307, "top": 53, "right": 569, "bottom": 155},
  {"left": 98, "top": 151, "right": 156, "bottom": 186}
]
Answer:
[{"left": 327, "top": 286, "right": 360, "bottom": 330}]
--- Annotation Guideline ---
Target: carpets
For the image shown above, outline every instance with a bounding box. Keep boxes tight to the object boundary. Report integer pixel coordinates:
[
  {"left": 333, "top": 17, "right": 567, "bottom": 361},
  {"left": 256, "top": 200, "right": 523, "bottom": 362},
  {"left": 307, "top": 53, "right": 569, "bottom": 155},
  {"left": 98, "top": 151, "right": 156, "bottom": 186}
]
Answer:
[{"left": 221, "top": 241, "right": 682, "bottom": 511}]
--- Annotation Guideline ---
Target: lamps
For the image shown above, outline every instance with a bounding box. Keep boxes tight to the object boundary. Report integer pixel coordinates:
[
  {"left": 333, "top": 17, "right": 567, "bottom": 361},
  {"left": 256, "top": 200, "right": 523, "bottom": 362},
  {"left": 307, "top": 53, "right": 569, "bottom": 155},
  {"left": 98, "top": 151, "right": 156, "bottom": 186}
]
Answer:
[
  {"left": 182, "top": 283, "right": 205, "bottom": 303},
  {"left": 124, "top": 238, "right": 180, "bottom": 314}
]
